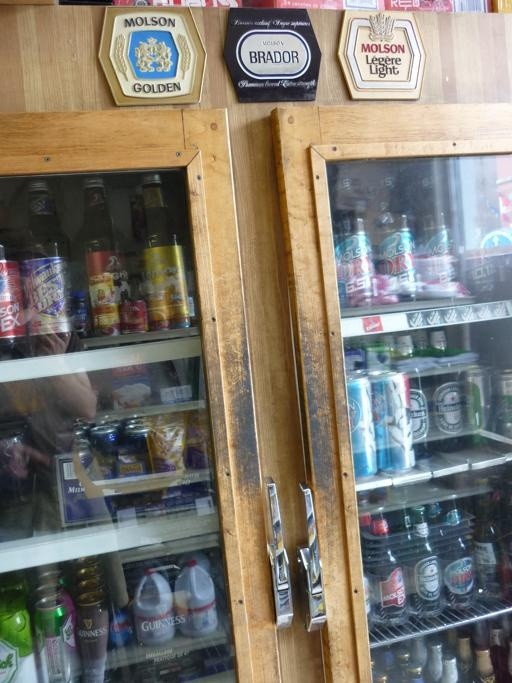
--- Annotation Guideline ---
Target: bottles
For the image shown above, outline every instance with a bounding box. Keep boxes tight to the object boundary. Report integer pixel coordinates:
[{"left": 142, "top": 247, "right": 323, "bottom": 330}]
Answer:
[
  {"left": 0, "top": 173, "right": 190, "bottom": 364},
  {"left": 353, "top": 490, "right": 512, "bottom": 633},
  {"left": 334, "top": 210, "right": 512, "bottom": 311},
  {"left": 378, "top": 325, "right": 470, "bottom": 459},
  {"left": 371, "top": 614, "right": 512, "bottom": 682}
]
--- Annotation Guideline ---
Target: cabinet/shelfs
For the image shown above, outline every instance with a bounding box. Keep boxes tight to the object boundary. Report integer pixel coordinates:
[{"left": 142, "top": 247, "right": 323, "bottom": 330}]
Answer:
[
  {"left": 270, "top": 101, "right": 512, "bottom": 683},
  {"left": 0, "top": 104, "right": 293, "bottom": 683}
]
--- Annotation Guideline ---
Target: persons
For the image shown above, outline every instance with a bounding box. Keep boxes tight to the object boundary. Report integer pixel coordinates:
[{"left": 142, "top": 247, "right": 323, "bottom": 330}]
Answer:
[{"left": 1, "top": 251, "right": 131, "bottom": 681}]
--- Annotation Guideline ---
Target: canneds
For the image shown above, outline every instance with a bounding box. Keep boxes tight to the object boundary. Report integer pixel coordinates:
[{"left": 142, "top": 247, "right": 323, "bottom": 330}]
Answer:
[
  {"left": 0, "top": 553, "right": 110, "bottom": 682},
  {"left": 72, "top": 412, "right": 150, "bottom": 526},
  {"left": 346, "top": 367, "right": 416, "bottom": 483},
  {"left": 461, "top": 364, "right": 512, "bottom": 453}
]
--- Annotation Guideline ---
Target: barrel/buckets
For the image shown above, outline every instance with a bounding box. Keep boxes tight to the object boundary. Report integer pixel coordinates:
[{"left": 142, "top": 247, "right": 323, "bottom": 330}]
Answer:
[
  {"left": 173, "top": 561, "right": 219, "bottom": 636},
  {"left": 132, "top": 569, "right": 175, "bottom": 645}
]
